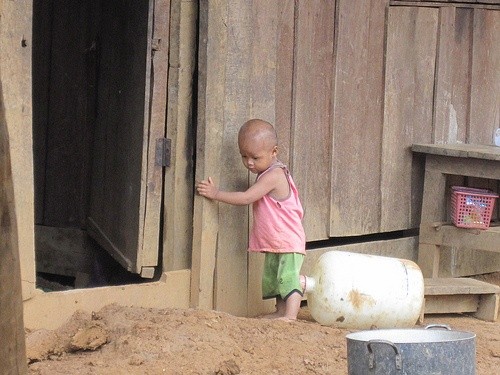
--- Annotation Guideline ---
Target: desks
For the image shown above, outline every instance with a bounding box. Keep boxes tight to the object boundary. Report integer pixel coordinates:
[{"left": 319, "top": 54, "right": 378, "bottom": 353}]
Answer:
[{"left": 410, "top": 142, "right": 500, "bottom": 278}]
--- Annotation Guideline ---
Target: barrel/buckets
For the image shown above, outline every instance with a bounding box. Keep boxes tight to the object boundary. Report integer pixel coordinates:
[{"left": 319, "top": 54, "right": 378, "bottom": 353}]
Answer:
[
  {"left": 301, "top": 251, "right": 424, "bottom": 329},
  {"left": 347, "top": 324, "right": 476, "bottom": 375}
]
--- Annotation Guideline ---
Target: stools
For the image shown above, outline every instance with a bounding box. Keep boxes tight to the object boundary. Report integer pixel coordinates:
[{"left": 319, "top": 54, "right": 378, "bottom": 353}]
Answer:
[{"left": 416, "top": 278, "right": 500, "bottom": 326}]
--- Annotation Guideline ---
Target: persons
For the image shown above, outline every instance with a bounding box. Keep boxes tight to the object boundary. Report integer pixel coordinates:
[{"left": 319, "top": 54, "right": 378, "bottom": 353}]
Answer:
[{"left": 197, "top": 119, "right": 305, "bottom": 321}]
